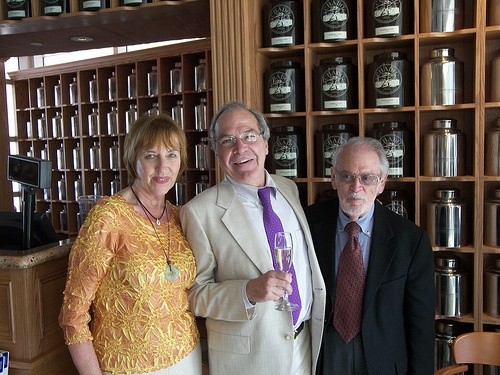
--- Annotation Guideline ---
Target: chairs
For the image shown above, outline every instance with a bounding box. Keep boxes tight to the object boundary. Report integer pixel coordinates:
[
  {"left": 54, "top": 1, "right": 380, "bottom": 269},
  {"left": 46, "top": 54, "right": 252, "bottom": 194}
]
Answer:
[{"left": 433, "top": 331, "right": 500, "bottom": 375}]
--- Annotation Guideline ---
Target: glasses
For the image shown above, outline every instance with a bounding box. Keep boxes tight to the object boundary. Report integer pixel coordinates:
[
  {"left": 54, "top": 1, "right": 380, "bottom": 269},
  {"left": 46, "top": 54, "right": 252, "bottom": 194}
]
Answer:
[
  {"left": 216, "top": 132, "right": 264, "bottom": 144},
  {"left": 339, "top": 172, "right": 382, "bottom": 185}
]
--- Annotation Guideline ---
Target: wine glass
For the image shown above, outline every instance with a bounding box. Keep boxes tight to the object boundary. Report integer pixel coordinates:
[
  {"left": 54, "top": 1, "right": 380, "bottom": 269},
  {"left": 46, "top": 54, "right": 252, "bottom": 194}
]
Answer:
[{"left": 273, "top": 232, "right": 301, "bottom": 311}]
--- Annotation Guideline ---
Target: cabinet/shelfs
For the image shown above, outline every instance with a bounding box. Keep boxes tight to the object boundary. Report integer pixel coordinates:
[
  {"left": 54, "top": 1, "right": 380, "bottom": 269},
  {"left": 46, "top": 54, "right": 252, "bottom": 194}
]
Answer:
[{"left": 0, "top": 0, "right": 500, "bottom": 375}]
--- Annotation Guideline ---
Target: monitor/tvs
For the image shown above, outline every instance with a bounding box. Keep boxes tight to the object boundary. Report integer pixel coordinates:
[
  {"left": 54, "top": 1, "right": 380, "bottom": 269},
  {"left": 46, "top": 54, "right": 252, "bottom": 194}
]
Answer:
[{"left": 0, "top": 211, "right": 59, "bottom": 252}]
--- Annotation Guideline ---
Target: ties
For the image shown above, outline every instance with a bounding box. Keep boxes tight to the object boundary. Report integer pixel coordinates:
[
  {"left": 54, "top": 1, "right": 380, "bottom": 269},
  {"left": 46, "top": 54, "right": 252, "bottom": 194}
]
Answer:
[
  {"left": 257, "top": 187, "right": 302, "bottom": 326},
  {"left": 332, "top": 222, "right": 365, "bottom": 344}
]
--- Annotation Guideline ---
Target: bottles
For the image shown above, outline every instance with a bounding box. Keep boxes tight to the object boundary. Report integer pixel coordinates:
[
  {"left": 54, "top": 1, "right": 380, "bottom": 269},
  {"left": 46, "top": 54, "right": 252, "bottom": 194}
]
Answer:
[
  {"left": 171, "top": 100, "right": 183, "bottom": 129},
  {"left": 484, "top": 189, "right": 500, "bottom": 247},
  {"left": 425, "top": 189, "right": 466, "bottom": 248},
  {"left": 372, "top": 121, "right": 407, "bottom": 179},
  {"left": 148, "top": 66, "right": 159, "bottom": 97},
  {"left": 195, "top": 174, "right": 209, "bottom": 197},
  {"left": 195, "top": 98, "right": 208, "bottom": 131},
  {"left": 432, "top": 258, "right": 468, "bottom": 317},
  {"left": 175, "top": 183, "right": 186, "bottom": 206},
  {"left": 195, "top": 59, "right": 206, "bottom": 91},
  {"left": 433, "top": 322, "right": 466, "bottom": 375},
  {"left": 4, "top": 0, "right": 149, "bottom": 19},
  {"left": 262, "top": 0, "right": 409, "bottom": 113},
  {"left": 321, "top": 124, "right": 353, "bottom": 177},
  {"left": 170, "top": 63, "right": 182, "bottom": 93},
  {"left": 272, "top": 125, "right": 301, "bottom": 179},
  {"left": 147, "top": 103, "right": 160, "bottom": 118},
  {"left": 486, "top": 259, "right": 500, "bottom": 317},
  {"left": 26, "top": 69, "right": 136, "bottom": 234},
  {"left": 419, "top": 1, "right": 500, "bottom": 178},
  {"left": 381, "top": 189, "right": 410, "bottom": 221},
  {"left": 195, "top": 137, "right": 210, "bottom": 169}
]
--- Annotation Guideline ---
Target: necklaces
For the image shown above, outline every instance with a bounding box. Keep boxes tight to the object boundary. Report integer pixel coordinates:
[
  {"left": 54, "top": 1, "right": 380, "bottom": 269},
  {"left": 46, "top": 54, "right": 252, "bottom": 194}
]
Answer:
[{"left": 131, "top": 186, "right": 178, "bottom": 281}]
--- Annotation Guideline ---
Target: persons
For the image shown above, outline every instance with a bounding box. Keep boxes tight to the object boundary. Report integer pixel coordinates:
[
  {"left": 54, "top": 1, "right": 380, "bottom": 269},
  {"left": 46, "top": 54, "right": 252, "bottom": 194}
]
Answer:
[
  {"left": 179, "top": 102, "right": 326, "bottom": 375},
  {"left": 58, "top": 114, "right": 202, "bottom": 375},
  {"left": 303, "top": 137, "right": 436, "bottom": 375}
]
